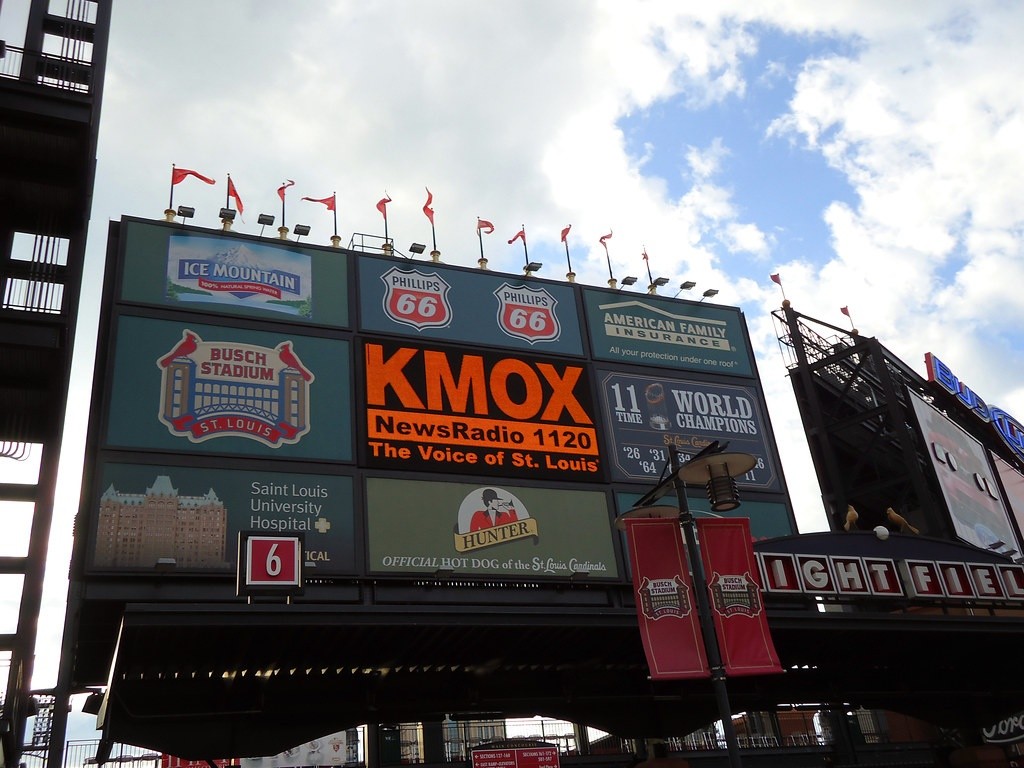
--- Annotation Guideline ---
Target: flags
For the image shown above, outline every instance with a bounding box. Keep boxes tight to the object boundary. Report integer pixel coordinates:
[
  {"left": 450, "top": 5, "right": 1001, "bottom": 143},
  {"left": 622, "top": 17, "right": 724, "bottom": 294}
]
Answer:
[{"left": 172, "top": 168, "right": 649, "bottom": 261}]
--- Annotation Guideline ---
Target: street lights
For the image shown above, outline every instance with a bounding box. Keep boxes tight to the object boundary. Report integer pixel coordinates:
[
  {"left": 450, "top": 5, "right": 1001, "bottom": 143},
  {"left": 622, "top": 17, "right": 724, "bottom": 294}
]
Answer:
[{"left": 631, "top": 438, "right": 762, "bottom": 748}]
